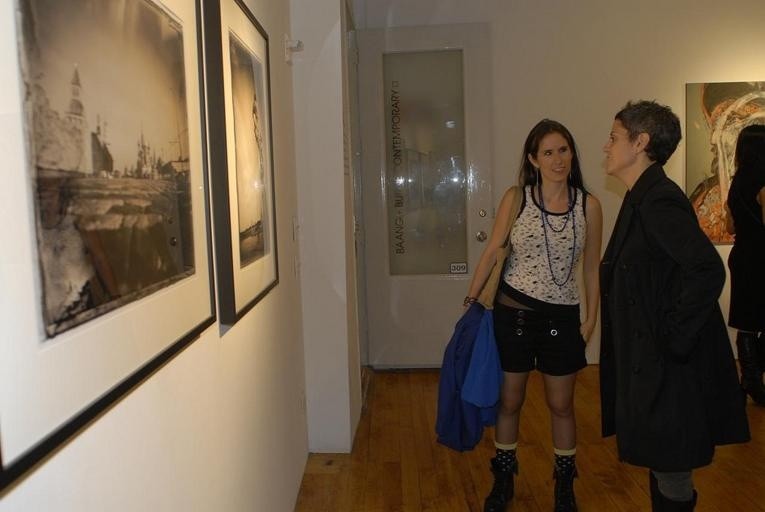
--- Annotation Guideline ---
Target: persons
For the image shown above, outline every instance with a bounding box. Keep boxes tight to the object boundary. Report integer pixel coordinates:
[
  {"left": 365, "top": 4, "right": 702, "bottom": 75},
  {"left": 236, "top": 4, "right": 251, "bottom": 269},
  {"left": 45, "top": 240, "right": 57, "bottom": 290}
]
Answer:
[
  {"left": 726, "top": 124, "right": 764, "bottom": 408},
  {"left": 598, "top": 99, "right": 750, "bottom": 511},
  {"left": 462, "top": 119, "right": 603, "bottom": 512}
]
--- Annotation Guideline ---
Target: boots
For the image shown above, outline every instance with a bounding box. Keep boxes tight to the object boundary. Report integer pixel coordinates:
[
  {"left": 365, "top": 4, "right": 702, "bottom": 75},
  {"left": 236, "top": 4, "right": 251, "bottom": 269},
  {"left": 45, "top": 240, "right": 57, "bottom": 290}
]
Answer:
[
  {"left": 553, "top": 465, "right": 579, "bottom": 512},
  {"left": 484, "top": 458, "right": 518, "bottom": 512}
]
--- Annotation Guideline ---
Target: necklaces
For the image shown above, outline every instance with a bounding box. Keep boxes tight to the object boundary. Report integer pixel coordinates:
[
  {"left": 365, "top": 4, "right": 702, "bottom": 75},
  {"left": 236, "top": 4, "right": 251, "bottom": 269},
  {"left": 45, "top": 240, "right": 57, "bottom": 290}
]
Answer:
[{"left": 537, "top": 184, "right": 576, "bottom": 286}]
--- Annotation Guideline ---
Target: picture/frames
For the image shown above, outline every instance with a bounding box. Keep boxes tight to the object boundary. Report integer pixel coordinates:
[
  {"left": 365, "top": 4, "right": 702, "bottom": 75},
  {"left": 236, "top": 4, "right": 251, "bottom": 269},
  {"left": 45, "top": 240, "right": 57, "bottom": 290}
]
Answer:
[
  {"left": 0, "top": 0, "right": 217, "bottom": 489},
  {"left": 201, "top": 0, "right": 280, "bottom": 325}
]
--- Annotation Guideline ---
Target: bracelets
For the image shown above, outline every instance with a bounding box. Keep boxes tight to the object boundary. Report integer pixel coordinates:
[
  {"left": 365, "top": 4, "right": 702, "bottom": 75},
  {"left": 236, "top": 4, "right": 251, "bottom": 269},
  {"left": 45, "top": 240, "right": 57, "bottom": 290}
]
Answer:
[{"left": 464, "top": 296, "right": 477, "bottom": 306}]
